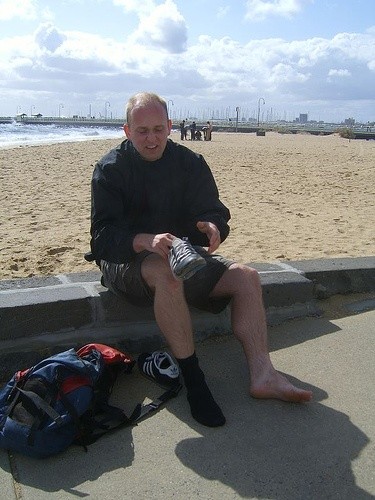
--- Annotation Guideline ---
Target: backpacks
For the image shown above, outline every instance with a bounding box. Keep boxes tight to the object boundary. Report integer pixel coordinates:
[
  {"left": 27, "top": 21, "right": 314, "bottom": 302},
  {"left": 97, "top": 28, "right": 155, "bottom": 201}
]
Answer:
[{"left": 0, "top": 345, "right": 143, "bottom": 458}]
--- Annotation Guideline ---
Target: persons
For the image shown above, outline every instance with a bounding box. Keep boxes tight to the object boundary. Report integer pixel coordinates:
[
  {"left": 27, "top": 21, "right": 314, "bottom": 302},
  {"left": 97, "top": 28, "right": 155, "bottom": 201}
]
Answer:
[
  {"left": 194, "top": 131, "right": 201, "bottom": 139},
  {"left": 179, "top": 120, "right": 185, "bottom": 140},
  {"left": 184, "top": 125, "right": 191, "bottom": 140},
  {"left": 82, "top": 92, "right": 312, "bottom": 427},
  {"left": 190, "top": 121, "right": 196, "bottom": 141},
  {"left": 202, "top": 121, "right": 212, "bottom": 141}
]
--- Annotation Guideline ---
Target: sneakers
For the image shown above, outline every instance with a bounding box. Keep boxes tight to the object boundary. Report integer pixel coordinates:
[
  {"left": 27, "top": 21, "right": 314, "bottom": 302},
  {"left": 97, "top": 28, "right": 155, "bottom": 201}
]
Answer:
[
  {"left": 138, "top": 350, "right": 184, "bottom": 391},
  {"left": 167, "top": 237, "right": 206, "bottom": 281}
]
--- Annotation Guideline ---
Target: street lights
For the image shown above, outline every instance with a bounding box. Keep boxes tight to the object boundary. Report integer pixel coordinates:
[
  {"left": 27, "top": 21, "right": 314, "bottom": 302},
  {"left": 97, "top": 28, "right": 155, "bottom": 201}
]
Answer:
[
  {"left": 31, "top": 105, "right": 35, "bottom": 118},
  {"left": 258, "top": 97, "right": 266, "bottom": 127},
  {"left": 168, "top": 100, "right": 174, "bottom": 113},
  {"left": 59, "top": 103, "right": 64, "bottom": 121},
  {"left": 17, "top": 105, "right": 21, "bottom": 115},
  {"left": 105, "top": 102, "right": 110, "bottom": 122}
]
